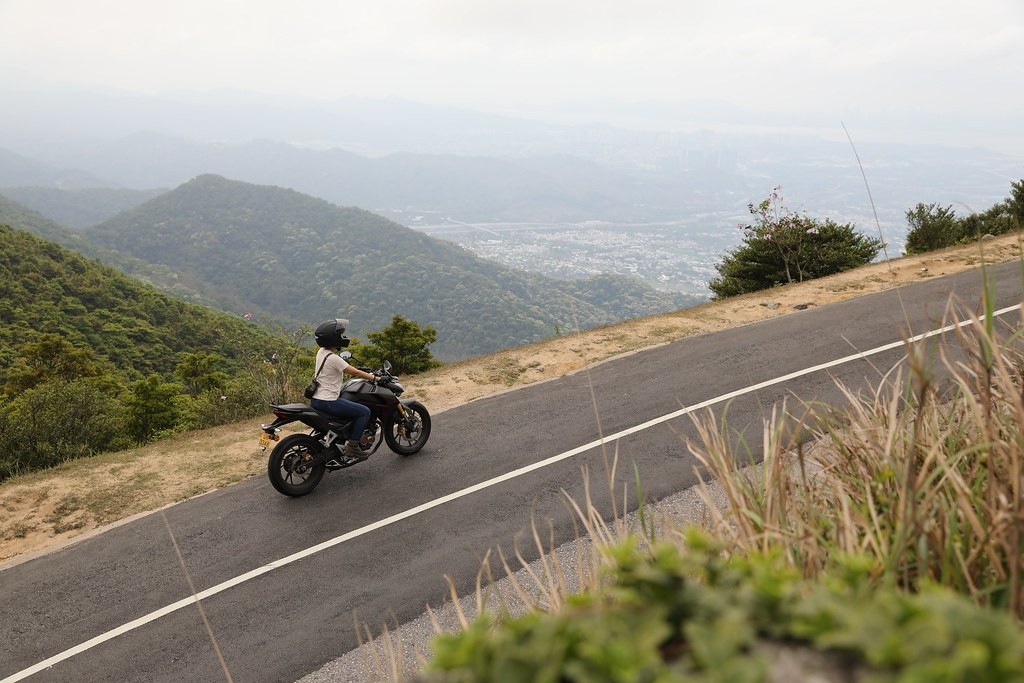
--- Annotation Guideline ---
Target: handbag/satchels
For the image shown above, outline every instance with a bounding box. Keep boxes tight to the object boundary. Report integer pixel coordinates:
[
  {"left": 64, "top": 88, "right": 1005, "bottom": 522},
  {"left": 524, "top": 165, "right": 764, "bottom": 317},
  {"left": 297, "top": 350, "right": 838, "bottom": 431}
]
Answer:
[{"left": 304, "top": 379, "right": 319, "bottom": 399}]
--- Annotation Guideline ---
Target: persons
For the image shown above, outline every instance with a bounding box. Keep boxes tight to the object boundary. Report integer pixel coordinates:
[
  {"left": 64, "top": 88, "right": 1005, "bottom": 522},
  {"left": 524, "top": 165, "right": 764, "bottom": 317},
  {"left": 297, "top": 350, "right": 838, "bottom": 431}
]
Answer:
[{"left": 310, "top": 319, "right": 391, "bottom": 458}]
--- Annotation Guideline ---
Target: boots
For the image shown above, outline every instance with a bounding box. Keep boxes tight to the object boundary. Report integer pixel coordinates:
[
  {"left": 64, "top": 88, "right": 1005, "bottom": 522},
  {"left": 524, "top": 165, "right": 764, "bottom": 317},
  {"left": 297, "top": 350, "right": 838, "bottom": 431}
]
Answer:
[{"left": 345, "top": 439, "right": 369, "bottom": 458}]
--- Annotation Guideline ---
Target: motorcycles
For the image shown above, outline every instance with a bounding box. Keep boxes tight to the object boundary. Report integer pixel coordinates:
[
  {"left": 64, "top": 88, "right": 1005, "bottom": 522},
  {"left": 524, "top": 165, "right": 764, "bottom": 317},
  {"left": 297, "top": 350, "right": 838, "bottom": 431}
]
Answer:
[{"left": 258, "top": 351, "right": 431, "bottom": 497}]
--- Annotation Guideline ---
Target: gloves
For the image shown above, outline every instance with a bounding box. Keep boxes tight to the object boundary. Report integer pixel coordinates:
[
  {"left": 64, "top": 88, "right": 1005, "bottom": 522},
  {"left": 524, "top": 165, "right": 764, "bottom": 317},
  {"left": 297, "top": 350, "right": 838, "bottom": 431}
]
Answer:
[{"left": 376, "top": 376, "right": 391, "bottom": 385}]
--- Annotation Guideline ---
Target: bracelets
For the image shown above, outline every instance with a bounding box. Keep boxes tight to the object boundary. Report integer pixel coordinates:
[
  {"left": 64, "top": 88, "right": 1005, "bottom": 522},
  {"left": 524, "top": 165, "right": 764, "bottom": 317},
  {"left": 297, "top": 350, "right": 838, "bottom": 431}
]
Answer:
[{"left": 371, "top": 376, "right": 375, "bottom": 382}]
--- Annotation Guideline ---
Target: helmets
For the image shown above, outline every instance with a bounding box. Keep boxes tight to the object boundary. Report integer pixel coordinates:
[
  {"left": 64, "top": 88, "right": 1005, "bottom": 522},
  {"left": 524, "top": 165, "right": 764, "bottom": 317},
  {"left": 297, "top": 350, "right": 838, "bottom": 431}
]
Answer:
[{"left": 315, "top": 320, "right": 350, "bottom": 351}]
